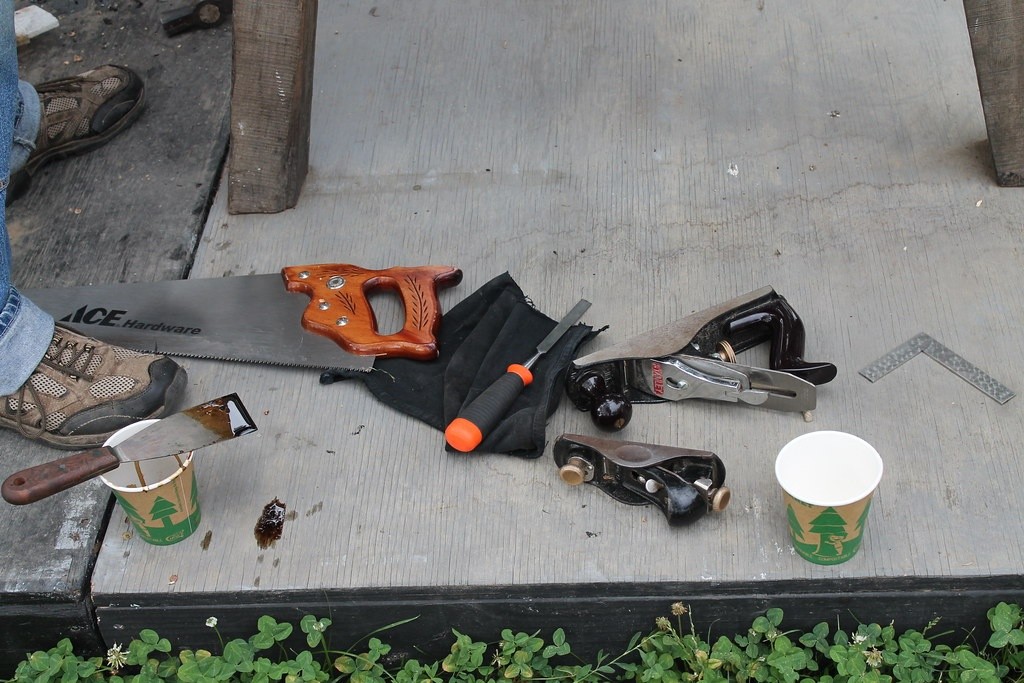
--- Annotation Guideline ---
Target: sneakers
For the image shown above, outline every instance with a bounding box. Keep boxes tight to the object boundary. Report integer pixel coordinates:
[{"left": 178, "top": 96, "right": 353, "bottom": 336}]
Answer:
[
  {"left": 4, "top": 63, "right": 148, "bottom": 208},
  {"left": 0, "top": 324, "right": 188, "bottom": 450}
]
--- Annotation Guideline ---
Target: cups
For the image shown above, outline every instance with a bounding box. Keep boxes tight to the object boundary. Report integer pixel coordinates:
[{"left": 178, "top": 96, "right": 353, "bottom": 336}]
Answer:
[
  {"left": 774, "top": 429, "right": 884, "bottom": 568},
  {"left": 96, "top": 418, "right": 202, "bottom": 546}
]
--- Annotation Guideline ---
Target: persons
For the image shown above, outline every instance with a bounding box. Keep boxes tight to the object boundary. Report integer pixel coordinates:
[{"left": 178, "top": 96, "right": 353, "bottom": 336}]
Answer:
[{"left": 0, "top": 0, "right": 188, "bottom": 452}]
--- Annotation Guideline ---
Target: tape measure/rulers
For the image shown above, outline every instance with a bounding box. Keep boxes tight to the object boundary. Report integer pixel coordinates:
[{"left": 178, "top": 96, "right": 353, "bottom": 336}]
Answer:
[{"left": 858, "top": 332, "right": 1017, "bottom": 406}]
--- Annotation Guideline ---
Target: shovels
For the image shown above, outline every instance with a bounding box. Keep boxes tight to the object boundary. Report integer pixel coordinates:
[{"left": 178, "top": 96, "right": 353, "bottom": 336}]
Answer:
[{"left": 0, "top": 391, "right": 258, "bottom": 506}]
